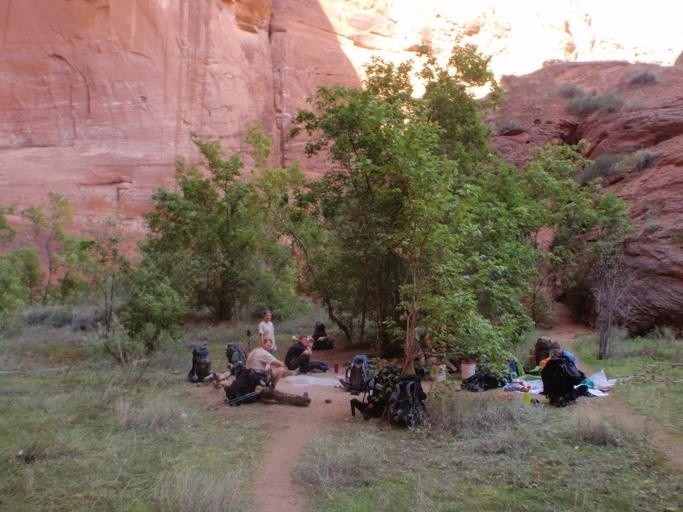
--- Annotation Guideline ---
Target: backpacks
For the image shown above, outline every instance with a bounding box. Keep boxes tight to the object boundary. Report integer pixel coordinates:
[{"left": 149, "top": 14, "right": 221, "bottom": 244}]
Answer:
[
  {"left": 350, "top": 363, "right": 402, "bottom": 420},
  {"left": 187, "top": 343, "right": 212, "bottom": 383},
  {"left": 524, "top": 335, "right": 553, "bottom": 376},
  {"left": 387, "top": 376, "right": 429, "bottom": 427},
  {"left": 539, "top": 355, "right": 589, "bottom": 408},
  {"left": 226, "top": 342, "right": 247, "bottom": 368},
  {"left": 460, "top": 369, "right": 500, "bottom": 393},
  {"left": 337, "top": 353, "right": 376, "bottom": 392}
]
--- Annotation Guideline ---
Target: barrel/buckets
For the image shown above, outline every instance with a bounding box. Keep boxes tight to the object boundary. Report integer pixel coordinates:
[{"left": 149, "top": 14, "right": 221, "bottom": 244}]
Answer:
[{"left": 460, "top": 359, "right": 477, "bottom": 381}]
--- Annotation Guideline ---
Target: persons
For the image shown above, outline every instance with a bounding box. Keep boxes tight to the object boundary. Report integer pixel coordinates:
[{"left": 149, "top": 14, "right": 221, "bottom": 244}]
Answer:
[
  {"left": 258, "top": 309, "right": 277, "bottom": 355},
  {"left": 285, "top": 335, "right": 312, "bottom": 375},
  {"left": 551, "top": 341, "right": 580, "bottom": 369},
  {"left": 245, "top": 338, "right": 286, "bottom": 394},
  {"left": 300, "top": 336, "right": 329, "bottom": 374},
  {"left": 313, "top": 320, "right": 333, "bottom": 350}
]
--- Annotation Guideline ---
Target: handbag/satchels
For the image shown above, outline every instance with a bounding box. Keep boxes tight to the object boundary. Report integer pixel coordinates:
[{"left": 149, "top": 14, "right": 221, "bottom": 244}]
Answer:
[{"left": 222, "top": 367, "right": 267, "bottom": 407}]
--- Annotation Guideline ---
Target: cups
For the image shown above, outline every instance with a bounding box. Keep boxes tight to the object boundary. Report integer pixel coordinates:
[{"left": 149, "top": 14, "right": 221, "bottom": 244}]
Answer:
[
  {"left": 524, "top": 392, "right": 532, "bottom": 406},
  {"left": 334, "top": 363, "right": 339, "bottom": 373},
  {"left": 304, "top": 391, "right": 309, "bottom": 397}
]
